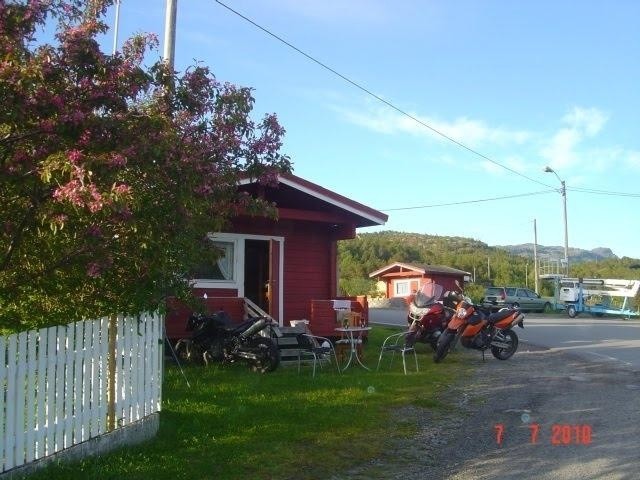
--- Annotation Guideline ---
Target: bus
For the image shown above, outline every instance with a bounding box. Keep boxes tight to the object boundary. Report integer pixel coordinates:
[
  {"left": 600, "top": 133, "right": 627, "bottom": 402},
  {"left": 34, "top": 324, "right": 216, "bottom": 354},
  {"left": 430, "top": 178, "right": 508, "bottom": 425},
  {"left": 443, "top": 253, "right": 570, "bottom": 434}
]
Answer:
[{"left": 560, "top": 278, "right": 640, "bottom": 304}]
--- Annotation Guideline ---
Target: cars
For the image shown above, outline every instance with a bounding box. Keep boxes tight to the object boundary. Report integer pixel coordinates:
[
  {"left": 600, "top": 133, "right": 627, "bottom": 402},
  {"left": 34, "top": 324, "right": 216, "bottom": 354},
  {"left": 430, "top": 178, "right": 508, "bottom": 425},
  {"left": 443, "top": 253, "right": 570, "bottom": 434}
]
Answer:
[{"left": 482, "top": 288, "right": 551, "bottom": 313}]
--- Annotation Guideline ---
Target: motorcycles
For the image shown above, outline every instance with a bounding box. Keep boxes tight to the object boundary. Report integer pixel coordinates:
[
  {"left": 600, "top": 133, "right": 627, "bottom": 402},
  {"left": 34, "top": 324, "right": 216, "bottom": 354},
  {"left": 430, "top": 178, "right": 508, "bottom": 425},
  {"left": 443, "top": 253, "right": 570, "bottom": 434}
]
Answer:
[
  {"left": 403, "top": 281, "right": 524, "bottom": 362},
  {"left": 175, "top": 293, "right": 279, "bottom": 373}
]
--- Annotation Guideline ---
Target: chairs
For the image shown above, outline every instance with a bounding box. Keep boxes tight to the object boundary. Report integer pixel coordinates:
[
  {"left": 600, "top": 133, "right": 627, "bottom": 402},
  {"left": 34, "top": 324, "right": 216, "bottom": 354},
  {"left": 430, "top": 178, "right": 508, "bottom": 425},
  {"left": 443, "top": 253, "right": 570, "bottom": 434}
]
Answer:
[{"left": 296, "top": 312, "right": 419, "bottom": 378}]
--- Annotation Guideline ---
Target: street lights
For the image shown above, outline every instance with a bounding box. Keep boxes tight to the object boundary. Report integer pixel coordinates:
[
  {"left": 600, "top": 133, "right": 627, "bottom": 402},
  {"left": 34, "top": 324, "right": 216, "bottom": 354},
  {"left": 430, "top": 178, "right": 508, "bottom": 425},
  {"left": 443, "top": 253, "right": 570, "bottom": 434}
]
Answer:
[{"left": 542, "top": 166, "right": 569, "bottom": 275}]
